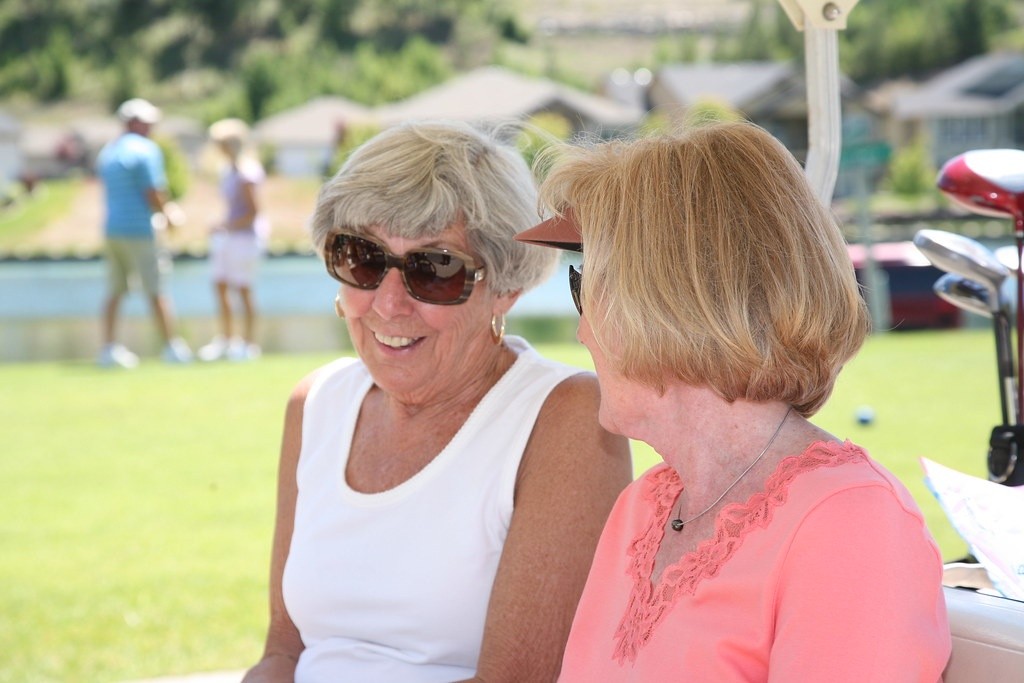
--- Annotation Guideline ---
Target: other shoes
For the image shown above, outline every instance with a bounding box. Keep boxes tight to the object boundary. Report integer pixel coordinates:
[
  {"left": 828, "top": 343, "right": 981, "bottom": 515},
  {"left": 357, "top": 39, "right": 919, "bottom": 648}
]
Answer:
[
  {"left": 98, "top": 342, "right": 139, "bottom": 370},
  {"left": 167, "top": 339, "right": 193, "bottom": 362},
  {"left": 200, "top": 334, "right": 263, "bottom": 361}
]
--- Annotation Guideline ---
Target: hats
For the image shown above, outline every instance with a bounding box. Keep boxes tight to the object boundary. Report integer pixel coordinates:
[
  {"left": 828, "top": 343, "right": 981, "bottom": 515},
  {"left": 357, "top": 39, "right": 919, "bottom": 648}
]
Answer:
[
  {"left": 119, "top": 98, "right": 161, "bottom": 126},
  {"left": 514, "top": 209, "right": 585, "bottom": 252}
]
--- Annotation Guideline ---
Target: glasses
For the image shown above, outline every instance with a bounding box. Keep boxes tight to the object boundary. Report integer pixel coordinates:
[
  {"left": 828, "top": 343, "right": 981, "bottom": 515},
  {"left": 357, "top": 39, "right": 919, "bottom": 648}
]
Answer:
[
  {"left": 569, "top": 264, "right": 583, "bottom": 315},
  {"left": 324, "top": 227, "right": 487, "bottom": 307}
]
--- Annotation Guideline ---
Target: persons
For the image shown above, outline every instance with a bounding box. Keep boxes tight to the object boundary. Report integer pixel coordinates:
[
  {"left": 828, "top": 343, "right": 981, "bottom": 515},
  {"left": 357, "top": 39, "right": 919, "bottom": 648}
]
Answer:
[
  {"left": 530, "top": 106, "right": 954, "bottom": 683},
  {"left": 241, "top": 123, "right": 633, "bottom": 683},
  {"left": 199, "top": 115, "right": 265, "bottom": 360},
  {"left": 99, "top": 99, "right": 190, "bottom": 368}
]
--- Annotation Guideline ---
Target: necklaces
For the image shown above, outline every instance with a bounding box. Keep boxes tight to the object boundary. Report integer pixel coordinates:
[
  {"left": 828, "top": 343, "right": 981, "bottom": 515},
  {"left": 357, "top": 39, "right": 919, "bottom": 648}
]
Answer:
[{"left": 671, "top": 405, "right": 794, "bottom": 531}]
